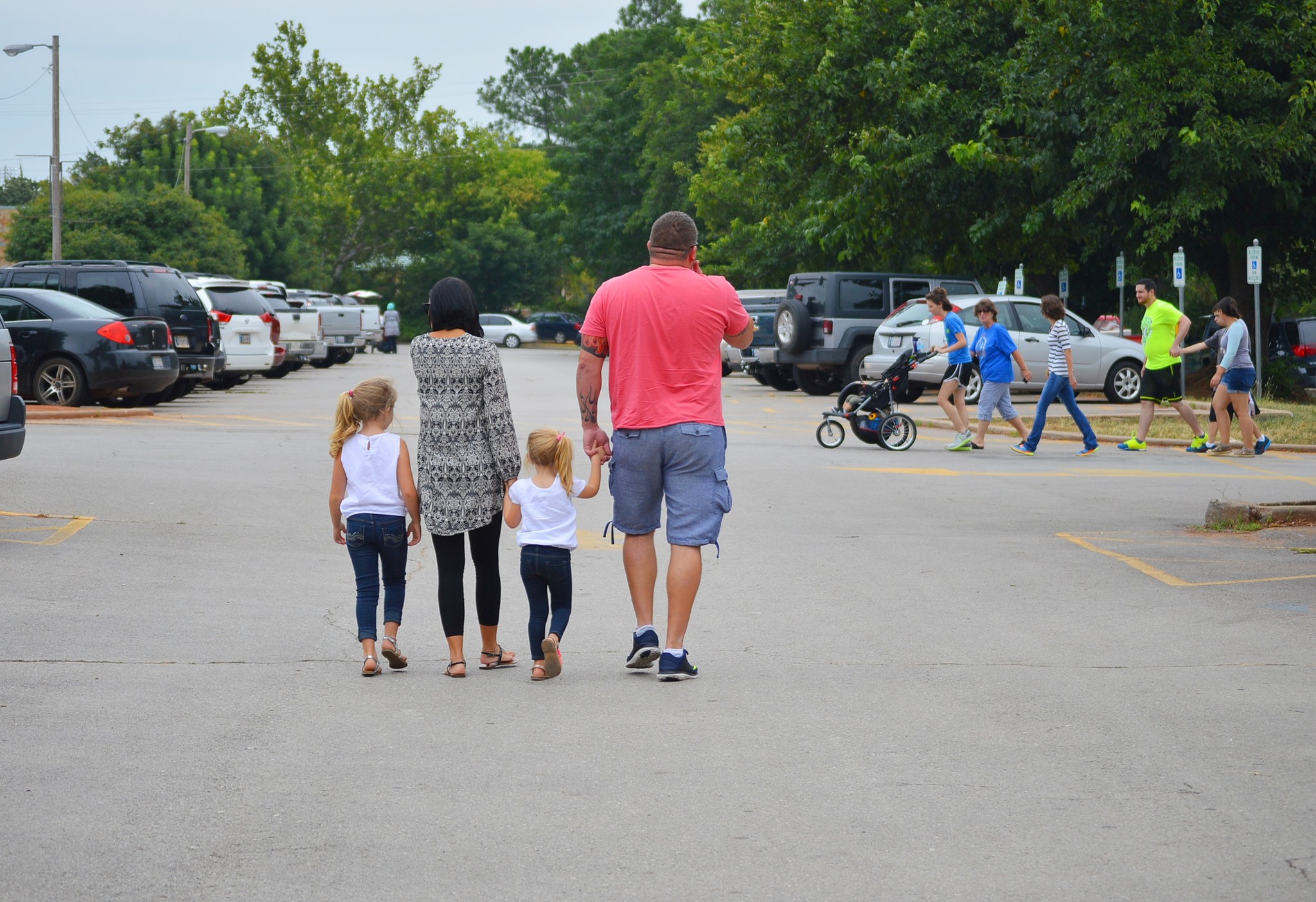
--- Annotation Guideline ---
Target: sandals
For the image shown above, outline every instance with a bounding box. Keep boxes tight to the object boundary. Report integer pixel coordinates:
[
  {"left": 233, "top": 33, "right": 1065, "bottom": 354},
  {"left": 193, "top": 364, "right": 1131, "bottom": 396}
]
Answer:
[
  {"left": 381, "top": 636, "right": 408, "bottom": 670},
  {"left": 442, "top": 659, "right": 467, "bottom": 677},
  {"left": 361, "top": 655, "right": 381, "bottom": 675},
  {"left": 479, "top": 645, "right": 519, "bottom": 670},
  {"left": 530, "top": 664, "right": 554, "bottom": 680},
  {"left": 541, "top": 636, "right": 563, "bottom": 679}
]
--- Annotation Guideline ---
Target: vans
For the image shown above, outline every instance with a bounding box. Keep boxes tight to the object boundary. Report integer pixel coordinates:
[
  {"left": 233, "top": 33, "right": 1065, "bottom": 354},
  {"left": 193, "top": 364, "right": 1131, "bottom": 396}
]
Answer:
[{"left": 717, "top": 288, "right": 789, "bottom": 380}]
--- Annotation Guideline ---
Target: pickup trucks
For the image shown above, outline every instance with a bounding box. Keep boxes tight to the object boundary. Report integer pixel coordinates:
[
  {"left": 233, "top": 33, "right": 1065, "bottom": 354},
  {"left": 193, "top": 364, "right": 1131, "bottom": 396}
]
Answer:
[
  {"left": 249, "top": 280, "right": 386, "bottom": 380},
  {"left": 725, "top": 305, "right": 803, "bottom": 393},
  {"left": 1090, "top": 315, "right": 1143, "bottom": 344}
]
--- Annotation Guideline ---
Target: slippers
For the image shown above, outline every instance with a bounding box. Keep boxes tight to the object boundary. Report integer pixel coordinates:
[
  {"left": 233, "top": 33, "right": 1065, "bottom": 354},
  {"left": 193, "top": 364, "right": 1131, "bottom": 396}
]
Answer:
[{"left": 970, "top": 441, "right": 984, "bottom": 449}]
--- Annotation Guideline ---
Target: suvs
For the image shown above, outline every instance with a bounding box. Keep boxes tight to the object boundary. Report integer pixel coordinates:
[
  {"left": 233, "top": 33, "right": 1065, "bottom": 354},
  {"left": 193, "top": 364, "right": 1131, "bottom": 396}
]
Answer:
[
  {"left": 0, "top": 258, "right": 226, "bottom": 404},
  {"left": 862, "top": 295, "right": 1144, "bottom": 404},
  {"left": 1267, "top": 316, "right": 1316, "bottom": 389},
  {"left": 1183, "top": 312, "right": 1228, "bottom": 377},
  {"left": 773, "top": 271, "right": 986, "bottom": 405}
]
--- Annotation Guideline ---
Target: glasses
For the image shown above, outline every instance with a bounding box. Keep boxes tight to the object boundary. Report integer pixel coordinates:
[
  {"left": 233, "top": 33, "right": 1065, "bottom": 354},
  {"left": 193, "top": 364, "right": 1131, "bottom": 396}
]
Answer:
[{"left": 976, "top": 308, "right": 990, "bottom": 315}]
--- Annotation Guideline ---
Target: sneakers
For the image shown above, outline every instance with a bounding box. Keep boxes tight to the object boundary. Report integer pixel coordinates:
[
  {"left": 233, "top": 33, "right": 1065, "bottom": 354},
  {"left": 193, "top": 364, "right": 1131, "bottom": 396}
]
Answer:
[
  {"left": 942, "top": 428, "right": 974, "bottom": 450},
  {"left": 1206, "top": 444, "right": 1232, "bottom": 455},
  {"left": 1228, "top": 447, "right": 1255, "bottom": 458},
  {"left": 656, "top": 649, "right": 698, "bottom": 681},
  {"left": 830, "top": 406, "right": 843, "bottom": 414},
  {"left": 1010, "top": 444, "right": 1035, "bottom": 456},
  {"left": 1255, "top": 435, "right": 1271, "bottom": 455},
  {"left": 1075, "top": 444, "right": 1100, "bottom": 456},
  {"left": 1186, "top": 433, "right": 1209, "bottom": 452},
  {"left": 1194, "top": 444, "right": 1216, "bottom": 452},
  {"left": 1117, "top": 430, "right": 1147, "bottom": 451},
  {"left": 624, "top": 629, "right": 661, "bottom": 669}
]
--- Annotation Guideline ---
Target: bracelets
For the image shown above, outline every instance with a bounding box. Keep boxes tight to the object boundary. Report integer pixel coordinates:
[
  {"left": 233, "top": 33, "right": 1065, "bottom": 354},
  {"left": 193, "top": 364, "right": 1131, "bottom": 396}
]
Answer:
[{"left": 1020, "top": 368, "right": 1029, "bottom": 371}]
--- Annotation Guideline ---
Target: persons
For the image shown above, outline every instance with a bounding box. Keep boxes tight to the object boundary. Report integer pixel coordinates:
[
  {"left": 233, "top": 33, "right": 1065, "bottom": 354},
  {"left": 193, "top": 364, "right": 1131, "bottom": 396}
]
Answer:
[
  {"left": 502, "top": 428, "right": 607, "bottom": 682},
  {"left": 969, "top": 297, "right": 1032, "bottom": 450},
  {"left": 925, "top": 287, "right": 973, "bottom": 450},
  {"left": 328, "top": 380, "right": 425, "bottom": 677},
  {"left": 1205, "top": 296, "right": 1257, "bottom": 458},
  {"left": 1115, "top": 278, "right": 1211, "bottom": 453},
  {"left": 576, "top": 212, "right": 754, "bottom": 679},
  {"left": 1173, "top": 302, "right": 1270, "bottom": 455},
  {"left": 1010, "top": 295, "right": 1101, "bottom": 456},
  {"left": 407, "top": 276, "right": 522, "bottom": 679},
  {"left": 837, "top": 375, "right": 895, "bottom": 416},
  {"left": 382, "top": 302, "right": 401, "bottom": 352}
]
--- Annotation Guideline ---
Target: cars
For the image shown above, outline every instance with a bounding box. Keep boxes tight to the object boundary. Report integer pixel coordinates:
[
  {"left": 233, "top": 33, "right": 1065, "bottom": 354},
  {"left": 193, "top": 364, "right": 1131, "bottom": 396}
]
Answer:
[
  {"left": 477, "top": 313, "right": 538, "bottom": 350},
  {"left": 0, "top": 312, "right": 27, "bottom": 462},
  {"left": 181, "top": 271, "right": 286, "bottom": 391},
  {"left": 0, "top": 286, "right": 180, "bottom": 407},
  {"left": 524, "top": 311, "right": 583, "bottom": 344}
]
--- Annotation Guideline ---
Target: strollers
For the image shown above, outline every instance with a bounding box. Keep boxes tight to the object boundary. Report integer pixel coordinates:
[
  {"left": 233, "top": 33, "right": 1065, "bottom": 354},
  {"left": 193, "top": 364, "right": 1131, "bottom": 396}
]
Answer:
[{"left": 816, "top": 346, "right": 942, "bottom": 453}]
